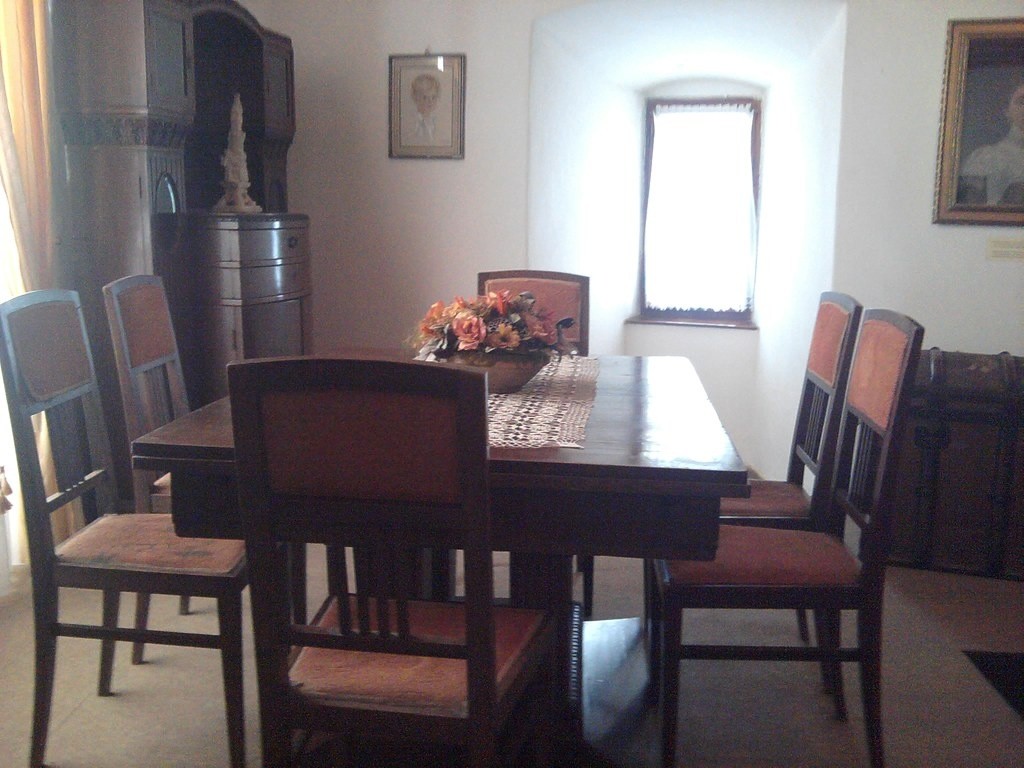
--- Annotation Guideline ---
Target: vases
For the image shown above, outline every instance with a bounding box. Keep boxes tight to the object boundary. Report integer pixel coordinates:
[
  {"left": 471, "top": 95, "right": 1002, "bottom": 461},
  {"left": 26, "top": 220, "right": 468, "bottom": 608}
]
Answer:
[{"left": 444, "top": 346, "right": 549, "bottom": 394}]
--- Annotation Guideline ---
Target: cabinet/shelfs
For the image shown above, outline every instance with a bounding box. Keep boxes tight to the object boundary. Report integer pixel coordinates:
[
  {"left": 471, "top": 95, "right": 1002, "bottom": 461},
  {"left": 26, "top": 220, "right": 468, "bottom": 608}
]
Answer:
[{"left": 48, "top": 0, "right": 313, "bottom": 515}]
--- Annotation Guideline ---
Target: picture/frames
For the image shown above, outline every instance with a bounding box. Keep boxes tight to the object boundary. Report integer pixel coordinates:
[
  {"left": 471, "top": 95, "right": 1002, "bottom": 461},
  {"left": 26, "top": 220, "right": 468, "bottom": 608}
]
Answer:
[
  {"left": 389, "top": 54, "right": 465, "bottom": 160},
  {"left": 932, "top": 18, "right": 1024, "bottom": 225}
]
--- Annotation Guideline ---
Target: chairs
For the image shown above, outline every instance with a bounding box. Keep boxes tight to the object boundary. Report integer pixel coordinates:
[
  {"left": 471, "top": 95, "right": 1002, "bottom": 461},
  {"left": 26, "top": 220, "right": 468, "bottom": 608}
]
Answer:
[
  {"left": 103, "top": 273, "right": 192, "bottom": 665},
  {"left": 648, "top": 309, "right": 925, "bottom": 768},
  {"left": 225, "top": 356, "right": 561, "bottom": 768},
  {"left": 640, "top": 292, "right": 864, "bottom": 695},
  {"left": 477, "top": 270, "right": 591, "bottom": 570},
  {"left": 0, "top": 289, "right": 264, "bottom": 768}
]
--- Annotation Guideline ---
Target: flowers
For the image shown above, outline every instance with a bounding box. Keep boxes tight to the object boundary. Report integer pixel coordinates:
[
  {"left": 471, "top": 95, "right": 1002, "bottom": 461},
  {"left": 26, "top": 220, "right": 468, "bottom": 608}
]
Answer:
[{"left": 404, "top": 289, "right": 582, "bottom": 371}]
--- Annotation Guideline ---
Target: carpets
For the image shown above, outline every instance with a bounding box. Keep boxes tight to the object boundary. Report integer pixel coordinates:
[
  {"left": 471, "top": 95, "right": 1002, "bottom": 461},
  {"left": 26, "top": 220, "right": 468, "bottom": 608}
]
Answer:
[{"left": 962, "top": 650, "right": 1024, "bottom": 721}]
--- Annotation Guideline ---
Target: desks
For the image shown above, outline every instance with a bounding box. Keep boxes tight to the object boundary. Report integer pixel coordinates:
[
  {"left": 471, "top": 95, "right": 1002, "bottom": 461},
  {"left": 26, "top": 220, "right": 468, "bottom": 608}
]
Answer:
[{"left": 131, "top": 348, "right": 752, "bottom": 768}]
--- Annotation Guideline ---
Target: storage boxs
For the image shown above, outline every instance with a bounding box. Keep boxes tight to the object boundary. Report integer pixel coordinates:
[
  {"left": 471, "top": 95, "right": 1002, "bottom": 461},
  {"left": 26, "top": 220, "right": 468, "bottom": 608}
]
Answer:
[{"left": 860, "top": 347, "right": 1023, "bottom": 580}]
